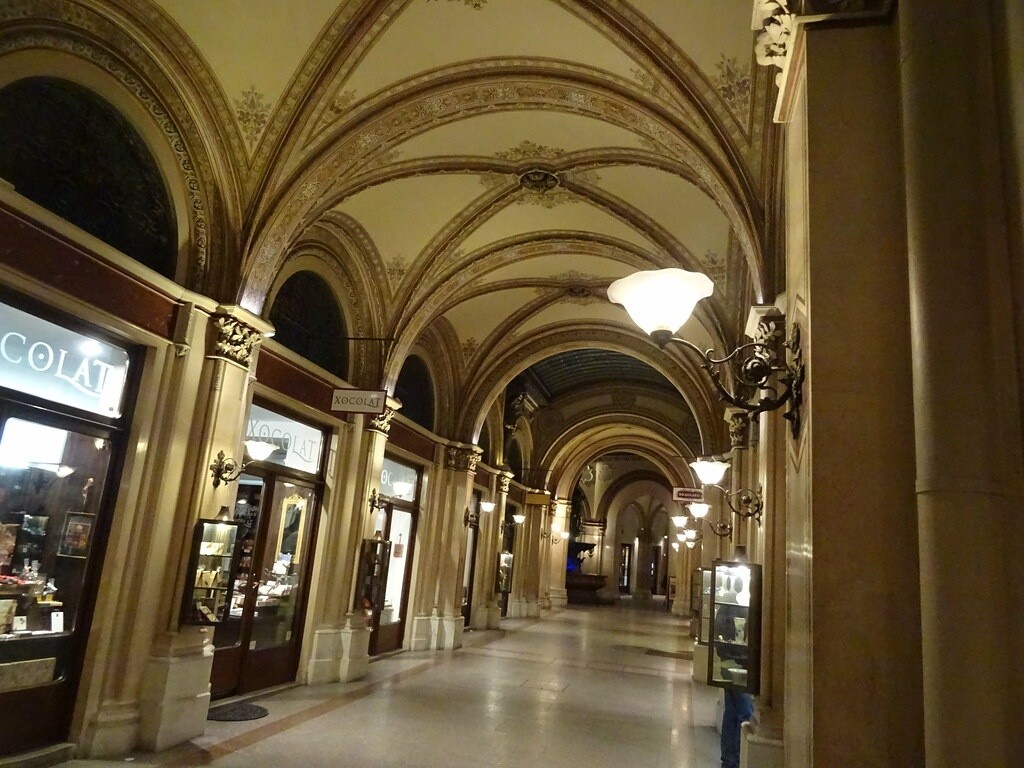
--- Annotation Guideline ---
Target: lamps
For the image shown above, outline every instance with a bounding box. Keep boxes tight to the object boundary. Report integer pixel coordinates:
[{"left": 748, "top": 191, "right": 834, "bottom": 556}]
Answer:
[
  {"left": 670, "top": 515, "right": 702, "bottom": 552},
  {"left": 541, "top": 524, "right": 569, "bottom": 545},
  {"left": 463, "top": 502, "right": 497, "bottom": 528},
  {"left": 210, "top": 440, "right": 280, "bottom": 488},
  {"left": 370, "top": 481, "right": 412, "bottom": 515},
  {"left": 684, "top": 502, "right": 733, "bottom": 544},
  {"left": 501, "top": 515, "right": 526, "bottom": 534},
  {"left": 689, "top": 460, "right": 763, "bottom": 527},
  {"left": 606, "top": 267, "right": 806, "bottom": 438}
]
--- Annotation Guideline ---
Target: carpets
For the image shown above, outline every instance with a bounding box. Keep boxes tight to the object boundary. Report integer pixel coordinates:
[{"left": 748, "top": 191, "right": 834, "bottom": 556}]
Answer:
[{"left": 207, "top": 703, "right": 268, "bottom": 721}]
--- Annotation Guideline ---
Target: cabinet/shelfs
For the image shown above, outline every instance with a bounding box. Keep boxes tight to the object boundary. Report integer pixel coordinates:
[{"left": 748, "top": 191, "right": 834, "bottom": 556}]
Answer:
[
  {"left": 179, "top": 520, "right": 245, "bottom": 626},
  {"left": 699, "top": 545, "right": 763, "bottom": 697},
  {"left": 353, "top": 531, "right": 392, "bottom": 612},
  {"left": 496, "top": 548, "right": 515, "bottom": 595}
]
